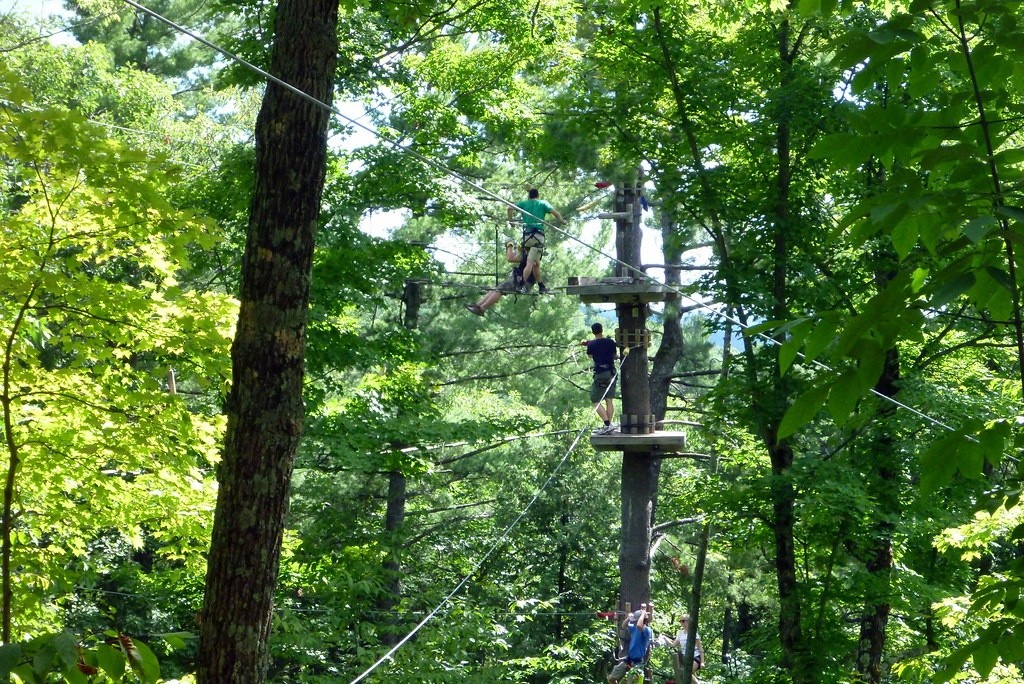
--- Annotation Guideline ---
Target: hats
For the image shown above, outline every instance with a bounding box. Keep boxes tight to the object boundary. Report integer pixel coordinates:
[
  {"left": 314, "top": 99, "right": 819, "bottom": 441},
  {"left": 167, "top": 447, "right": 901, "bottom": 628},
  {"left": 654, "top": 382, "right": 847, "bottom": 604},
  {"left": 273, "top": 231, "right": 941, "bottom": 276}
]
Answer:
[{"left": 629, "top": 610, "right": 648, "bottom": 623}]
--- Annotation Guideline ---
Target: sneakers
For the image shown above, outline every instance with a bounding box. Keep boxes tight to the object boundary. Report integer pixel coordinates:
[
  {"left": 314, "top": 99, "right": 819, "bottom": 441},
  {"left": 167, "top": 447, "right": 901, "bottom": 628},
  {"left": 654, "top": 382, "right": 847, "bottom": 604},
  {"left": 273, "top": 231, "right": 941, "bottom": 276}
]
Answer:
[
  {"left": 599, "top": 424, "right": 614, "bottom": 436},
  {"left": 592, "top": 427, "right": 606, "bottom": 432}
]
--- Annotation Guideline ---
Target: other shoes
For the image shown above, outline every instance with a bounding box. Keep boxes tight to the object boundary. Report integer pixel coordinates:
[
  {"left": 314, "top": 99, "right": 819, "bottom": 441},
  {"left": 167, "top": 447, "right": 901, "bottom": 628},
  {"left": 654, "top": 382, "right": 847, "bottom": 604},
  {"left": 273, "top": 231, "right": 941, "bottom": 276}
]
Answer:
[
  {"left": 539, "top": 284, "right": 551, "bottom": 294},
  {"left": 513, "top": 284, "right": 527, "bottom": 293},
  {"left": 464, "top": 302, "right": 483, "bottom": 318}
]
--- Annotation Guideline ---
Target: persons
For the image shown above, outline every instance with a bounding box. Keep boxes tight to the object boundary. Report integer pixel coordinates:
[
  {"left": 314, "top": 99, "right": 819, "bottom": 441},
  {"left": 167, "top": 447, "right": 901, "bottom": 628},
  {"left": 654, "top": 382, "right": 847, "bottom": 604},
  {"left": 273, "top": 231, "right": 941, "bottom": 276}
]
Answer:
[
  {"left": 608, "top": 610, "right": 652, "bottom": 684},
  {"left": 663, "top": 614, "right": 706, "bottom": 684},
  {"left": 508, "top": 188, "right": 568, "bottom": 294},
  {"left": 587, "top": 323, "right": 621, "bottom": 434},
  {"left": 464, "top": 241, "right": 543, "bottom": 317}
]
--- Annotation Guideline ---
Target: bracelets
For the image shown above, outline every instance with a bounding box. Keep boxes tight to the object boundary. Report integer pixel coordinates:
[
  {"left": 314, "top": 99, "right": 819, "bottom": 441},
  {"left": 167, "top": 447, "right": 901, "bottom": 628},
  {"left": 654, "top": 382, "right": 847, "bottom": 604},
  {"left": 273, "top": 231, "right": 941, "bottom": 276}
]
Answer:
[{"left": 507, "top": 242, "right": 514, "bottom": 247}]
